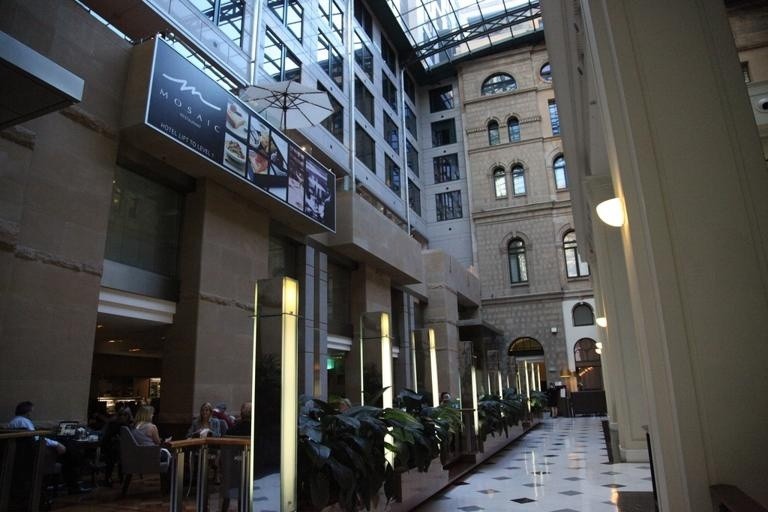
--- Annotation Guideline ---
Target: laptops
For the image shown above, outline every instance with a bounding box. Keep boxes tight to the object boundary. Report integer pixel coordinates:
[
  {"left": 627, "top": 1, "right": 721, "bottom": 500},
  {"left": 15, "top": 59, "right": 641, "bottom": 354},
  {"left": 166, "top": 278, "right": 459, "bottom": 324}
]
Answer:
[{"left": 56, "top": 421, "right": 79, "bottom": 439}]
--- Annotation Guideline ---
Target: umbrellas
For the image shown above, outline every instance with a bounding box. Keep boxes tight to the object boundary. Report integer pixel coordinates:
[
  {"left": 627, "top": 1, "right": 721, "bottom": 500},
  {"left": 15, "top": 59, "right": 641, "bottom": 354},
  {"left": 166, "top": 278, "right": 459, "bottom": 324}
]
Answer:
[{"left": 240, "top": 79, "right": 338, "bottom": 136}]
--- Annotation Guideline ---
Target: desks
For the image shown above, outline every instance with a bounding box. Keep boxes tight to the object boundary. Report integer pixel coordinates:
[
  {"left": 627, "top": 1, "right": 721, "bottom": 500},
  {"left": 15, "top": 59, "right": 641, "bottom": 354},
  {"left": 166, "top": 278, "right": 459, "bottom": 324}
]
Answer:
[{"left": 59, "top": 440, "right": 97, "bottom": 495}]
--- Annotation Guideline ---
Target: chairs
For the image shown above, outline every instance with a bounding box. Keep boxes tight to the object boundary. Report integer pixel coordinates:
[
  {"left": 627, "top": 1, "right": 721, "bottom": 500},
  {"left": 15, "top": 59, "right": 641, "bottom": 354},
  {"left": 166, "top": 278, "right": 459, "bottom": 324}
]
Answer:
[
  {"left": 5, "top": 427, "right": 61, "bottom": 498},
  {"left": 80, "top": 438, "right": 119, "bottom": 489},
  {"left": 118, "top": 426, "right": 161, "bottom": 495},
  {"left": 220, "top": 419, "right": 229, "bottom": 434}
]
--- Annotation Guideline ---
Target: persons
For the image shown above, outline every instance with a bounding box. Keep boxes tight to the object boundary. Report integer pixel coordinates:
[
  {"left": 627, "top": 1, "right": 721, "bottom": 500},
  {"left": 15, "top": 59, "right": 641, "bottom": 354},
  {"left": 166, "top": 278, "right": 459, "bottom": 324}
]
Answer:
[
  {"left": 5, "top": 395, "right": 354, "bottom": 502},
  {"left": 547, "top": 382, "right": 559, "bottom": 418}
]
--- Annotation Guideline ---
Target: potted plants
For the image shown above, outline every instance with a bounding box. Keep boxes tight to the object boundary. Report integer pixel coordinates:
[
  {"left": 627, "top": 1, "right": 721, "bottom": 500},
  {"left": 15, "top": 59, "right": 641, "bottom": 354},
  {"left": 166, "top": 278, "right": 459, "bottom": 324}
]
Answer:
[{"left": 297, "top": 386, "right": 553, "bottom": 512}]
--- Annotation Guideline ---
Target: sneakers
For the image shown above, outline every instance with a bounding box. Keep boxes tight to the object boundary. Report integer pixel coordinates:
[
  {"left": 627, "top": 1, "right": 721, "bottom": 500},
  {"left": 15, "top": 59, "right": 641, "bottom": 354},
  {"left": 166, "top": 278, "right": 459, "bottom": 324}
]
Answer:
[{"left": 45, "top": 476, "right": 114, "bottom": 495}]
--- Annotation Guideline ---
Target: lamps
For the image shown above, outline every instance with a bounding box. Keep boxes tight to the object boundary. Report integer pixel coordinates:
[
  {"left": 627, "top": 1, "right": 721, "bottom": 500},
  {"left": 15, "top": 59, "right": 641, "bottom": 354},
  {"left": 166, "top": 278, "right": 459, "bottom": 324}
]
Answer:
[
  {"left": 595, "top": 318, "right": 607, "bottom": 328},
  {"left": 560, "top": 369, "right": 572, "bottom": 397},
  {"left": 595, "top": 343, "right": 603, "bottom": 348},
  {"left": 596, "top": 197, "right": 625, "bottom": 227},
  {"left": 595, "top": 349, "right": 601, "bottom": 354}
]
